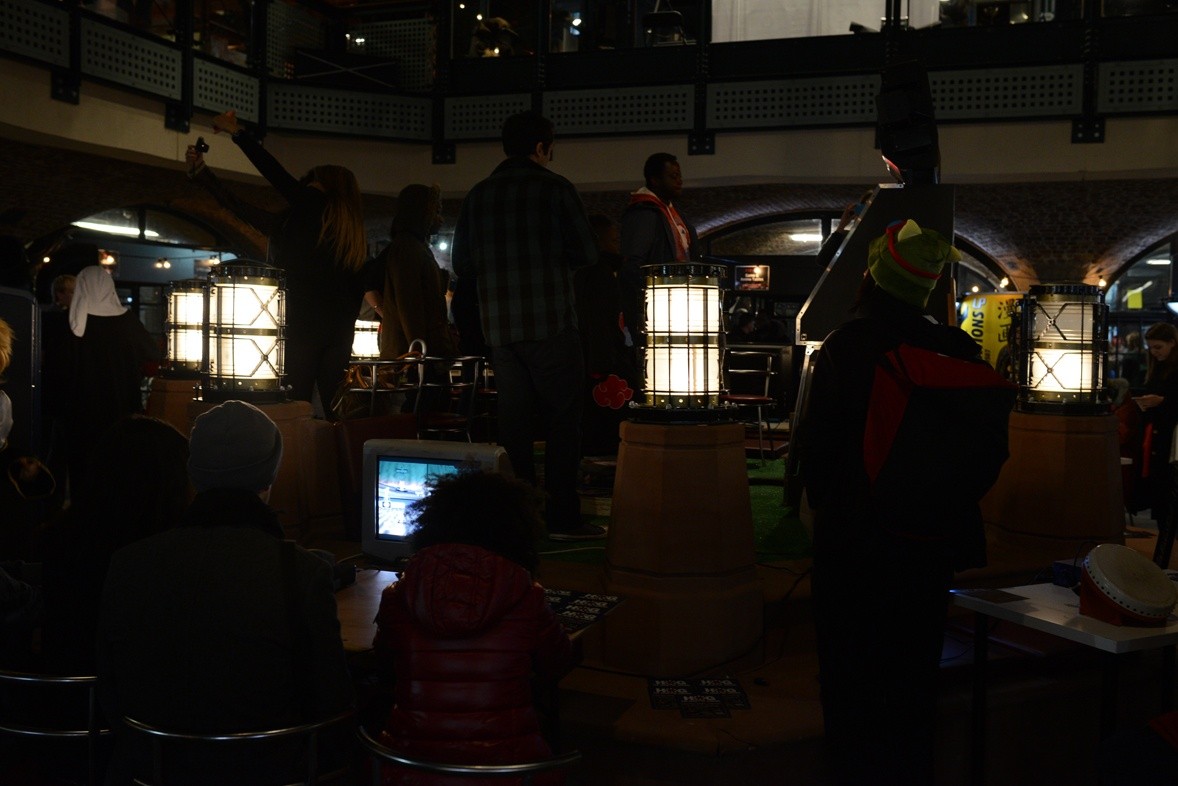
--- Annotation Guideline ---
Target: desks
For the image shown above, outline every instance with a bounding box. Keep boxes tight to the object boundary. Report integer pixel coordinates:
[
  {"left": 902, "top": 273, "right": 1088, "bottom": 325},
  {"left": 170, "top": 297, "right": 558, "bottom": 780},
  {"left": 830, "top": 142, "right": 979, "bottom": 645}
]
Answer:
[{"left": 946, "top": 582, "right": 1177, "bottom": 785}]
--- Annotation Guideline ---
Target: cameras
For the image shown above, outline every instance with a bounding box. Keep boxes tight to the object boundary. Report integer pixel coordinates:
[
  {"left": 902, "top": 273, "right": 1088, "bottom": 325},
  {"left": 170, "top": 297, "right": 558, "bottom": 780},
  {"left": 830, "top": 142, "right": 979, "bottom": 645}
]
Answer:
[{"left": 194, "top": 138, "right": 208, "bottom": 153}]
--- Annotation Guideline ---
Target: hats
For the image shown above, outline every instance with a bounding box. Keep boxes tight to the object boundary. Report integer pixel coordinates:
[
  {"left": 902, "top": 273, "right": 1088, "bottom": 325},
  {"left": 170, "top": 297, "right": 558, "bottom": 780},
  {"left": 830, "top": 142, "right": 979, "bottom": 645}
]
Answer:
[
  {"left": 190, "top": 398, "right": 284, "bottom": 492},
  {"left": 870, "top": 220, "right": 961, "bottom": 308}
]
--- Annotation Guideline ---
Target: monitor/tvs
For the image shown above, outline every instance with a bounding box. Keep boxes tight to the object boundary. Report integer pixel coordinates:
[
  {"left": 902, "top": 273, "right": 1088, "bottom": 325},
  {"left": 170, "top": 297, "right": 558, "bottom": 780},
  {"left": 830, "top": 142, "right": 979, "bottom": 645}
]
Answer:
[{"left": 361, "top": 437, "right": 516, "bottom": 571}]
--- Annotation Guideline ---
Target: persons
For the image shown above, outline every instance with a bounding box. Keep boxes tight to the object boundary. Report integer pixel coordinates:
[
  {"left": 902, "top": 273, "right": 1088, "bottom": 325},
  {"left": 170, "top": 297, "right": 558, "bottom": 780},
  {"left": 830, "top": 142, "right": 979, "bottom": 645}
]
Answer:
[
  {"left": 1127, "top": 325, "right": 1178, "bottom": 571},
  {"left": 451, "top": 109, "right": 611, "bottom": 541},
  {"left": 1122, "top": 331, "right": 1150, "bottom": 386},
  {"left": 730, "top": 313, "right": 759, "bottom": 343},
  {"left": 90, "top": 396, "right": 358, "bottom": 786},
  {"left": 814, "top": 190, "right": 875, "bottom": 269},
  {"left": 364, "top": 467, "right": 572, "bottom": 785},
  {"left": 378, "top": 180, "right": 455, "bottom": 360},
  {"left": 577, "top": 212, "right": 642, "bottom": 403},
  {"left": 783, "top": 215, "right": 1017, "bottom": 786},
  {"left": 620, "top": 150, "right": 695, "bottom": 397},
  {"left": 0, "top": 107, "right": 372, "bottom": 423},
  {"left": 37, "top": 413, "right": 195, "bottom": 786}
]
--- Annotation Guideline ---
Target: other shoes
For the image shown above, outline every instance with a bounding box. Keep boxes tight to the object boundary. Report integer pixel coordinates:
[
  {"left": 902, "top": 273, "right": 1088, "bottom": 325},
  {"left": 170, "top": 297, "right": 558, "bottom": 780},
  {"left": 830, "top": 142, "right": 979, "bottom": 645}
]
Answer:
[{"left": 548, "top": 519, "right": 609, "bottom": 542}]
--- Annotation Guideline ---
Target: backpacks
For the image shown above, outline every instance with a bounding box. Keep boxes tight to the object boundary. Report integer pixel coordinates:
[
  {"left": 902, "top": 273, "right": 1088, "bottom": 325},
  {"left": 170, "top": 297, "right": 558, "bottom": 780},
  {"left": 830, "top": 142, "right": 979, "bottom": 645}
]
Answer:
[{"left": 864, "top": 339, "right": 1016, "bottom": 547}]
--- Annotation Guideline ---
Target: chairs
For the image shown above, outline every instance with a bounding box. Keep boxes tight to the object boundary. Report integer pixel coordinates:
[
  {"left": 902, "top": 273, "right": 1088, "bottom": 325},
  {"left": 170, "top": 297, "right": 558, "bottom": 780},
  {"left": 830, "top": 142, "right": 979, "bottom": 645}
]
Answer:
[
  {"left": 0, "top": 667, "right": 112, "bottom": 786},
  {"left": 108, "top": 702, "right": 356, "bottom": 786},
  {"left": 354, "top": 721, "right": 583, "bottom": 786}
]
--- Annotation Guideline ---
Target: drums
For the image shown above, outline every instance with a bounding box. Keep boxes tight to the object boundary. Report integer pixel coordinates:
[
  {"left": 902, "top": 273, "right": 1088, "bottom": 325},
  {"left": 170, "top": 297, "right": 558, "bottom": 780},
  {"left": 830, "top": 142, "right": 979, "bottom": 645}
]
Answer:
[{"left": 1078, "top": 541, "right": 1178, "bottom": 628}]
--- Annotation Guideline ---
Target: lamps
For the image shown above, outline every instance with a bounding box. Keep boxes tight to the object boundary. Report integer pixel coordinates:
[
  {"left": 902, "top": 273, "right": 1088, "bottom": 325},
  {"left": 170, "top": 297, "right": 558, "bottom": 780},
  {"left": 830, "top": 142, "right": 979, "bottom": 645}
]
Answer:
[
  {"left": 628, "top": 259, "right": 738, "bottom": 422},
  {"left": 152, "top": 279, "right": 203, "bottom": 378},
  {"left": 1011, "top": 286, "right": 1113, "bottom": 416},
  {"left": 194, "top": 258, "right": 292, "bottom": 407}
]
